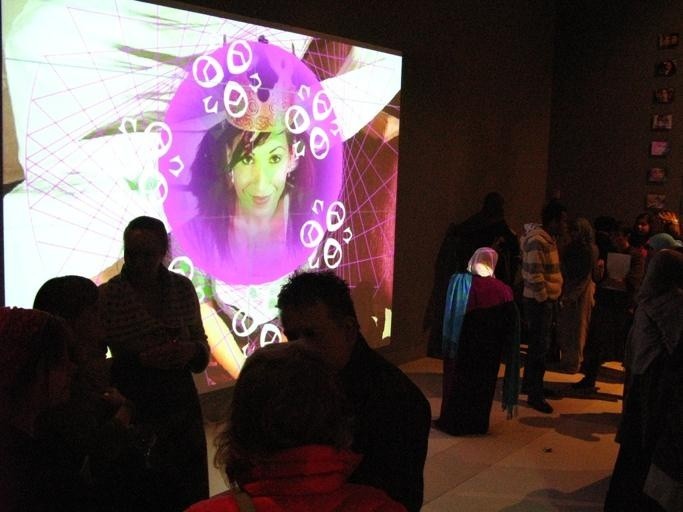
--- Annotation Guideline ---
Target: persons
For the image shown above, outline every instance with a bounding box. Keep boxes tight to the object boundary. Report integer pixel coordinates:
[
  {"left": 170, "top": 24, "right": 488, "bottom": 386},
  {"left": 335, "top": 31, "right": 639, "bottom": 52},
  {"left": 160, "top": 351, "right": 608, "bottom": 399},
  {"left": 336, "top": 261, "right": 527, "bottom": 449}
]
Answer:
[{"left": 171, "top": 34, "right": 336, "bottom": 388}]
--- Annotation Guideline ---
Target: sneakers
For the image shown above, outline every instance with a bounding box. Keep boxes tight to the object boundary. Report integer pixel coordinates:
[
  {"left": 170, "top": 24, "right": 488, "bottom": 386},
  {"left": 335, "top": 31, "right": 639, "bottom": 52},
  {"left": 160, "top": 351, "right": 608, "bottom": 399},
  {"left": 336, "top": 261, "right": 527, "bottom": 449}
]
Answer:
[
  {"left": 520, "top": 383, "right": 562, "bottom": 413},
  {"left": 559, "top": 384, "right": 600, "bottom": 394}
]
